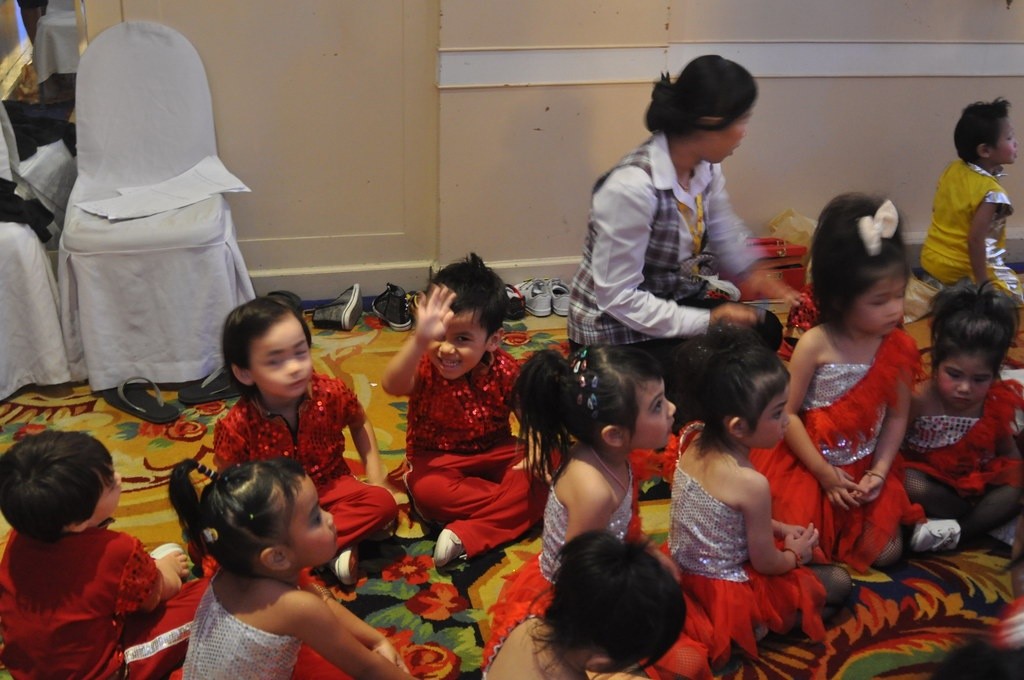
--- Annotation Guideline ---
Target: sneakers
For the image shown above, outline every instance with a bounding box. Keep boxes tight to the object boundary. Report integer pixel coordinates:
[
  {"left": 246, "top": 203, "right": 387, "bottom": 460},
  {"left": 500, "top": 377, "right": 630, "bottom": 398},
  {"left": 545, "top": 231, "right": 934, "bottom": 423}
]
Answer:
[
  {"left": 502, "top": 284, "right": 526, "bottom": 319},
  {"left": 407, "top": 290, "right": 423, "bottom": 320},
  {"left": 910, "top": 519, "right": 961, "bottom": 555},
  {"left": 514, "top": 278, "right": 553, "bottom": 317},
  {"left": 985, "top": 515, "right": 1021, "bottom": 547},
  {"left": 545, "top": 277, "right": 572, "bottom": 317}
]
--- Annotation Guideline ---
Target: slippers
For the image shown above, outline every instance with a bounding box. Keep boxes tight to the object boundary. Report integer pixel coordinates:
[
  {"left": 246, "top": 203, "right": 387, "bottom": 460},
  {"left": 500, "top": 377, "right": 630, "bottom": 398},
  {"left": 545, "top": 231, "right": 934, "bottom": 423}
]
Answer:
[
  {"left": 178, "top": 361, "right": 241, "bottom": 404},
  {"left": 106, "top": 375, "right": 181, "bottom": 423}
]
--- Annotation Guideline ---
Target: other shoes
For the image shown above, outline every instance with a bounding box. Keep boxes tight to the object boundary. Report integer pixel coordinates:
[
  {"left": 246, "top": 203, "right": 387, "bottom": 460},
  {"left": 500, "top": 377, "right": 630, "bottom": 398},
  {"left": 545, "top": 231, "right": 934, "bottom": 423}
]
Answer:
[
  {"left": 151, "top": 543, "right": 185, "bottom": 561},
  {"left": 327, "top": 541, "right": 358, "bottom": 587},
  {"left": 433, "top": 528, "right": 464, "bottom": 567}
]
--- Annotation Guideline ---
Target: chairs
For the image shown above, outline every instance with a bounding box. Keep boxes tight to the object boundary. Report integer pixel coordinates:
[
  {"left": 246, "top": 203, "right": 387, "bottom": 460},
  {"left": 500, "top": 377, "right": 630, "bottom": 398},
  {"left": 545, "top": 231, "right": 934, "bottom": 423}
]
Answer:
[
  {"left": 0, "top": 99, "right": 72, "bottom": 404},
  {"left": 33, "top": 0, "right": 80, "bottom": 100},
  {"left": 56, "top": 21, "right": 255, "bottom": 392}
]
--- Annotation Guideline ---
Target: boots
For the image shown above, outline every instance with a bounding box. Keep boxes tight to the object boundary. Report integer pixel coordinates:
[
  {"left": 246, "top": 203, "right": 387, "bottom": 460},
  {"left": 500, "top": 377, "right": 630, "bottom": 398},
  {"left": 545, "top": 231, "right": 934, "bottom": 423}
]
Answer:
[
  {"left": 311, "top": 283, "right": 363, "bottom": 331},
  {"left": 370, "top": 283, "right": 412, "bottom": 332}
]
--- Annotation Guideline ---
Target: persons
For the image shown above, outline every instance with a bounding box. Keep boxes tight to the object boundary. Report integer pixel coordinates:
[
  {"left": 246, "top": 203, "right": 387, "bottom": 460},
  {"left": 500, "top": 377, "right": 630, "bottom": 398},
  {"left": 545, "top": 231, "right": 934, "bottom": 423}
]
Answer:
[
  {"left": 747, "top": 190, "right": 928, "bottom": 576},
  {"left": 565, "top": 53, "right": 807, "bottom": 434},
  {"left": 211, "top": 294, "right": 399, "bottom": 587},
  {"left": 481, "top": 531, "right": 683, "bottom": 680},
  {"left": 921, "top": 98, "right": 1023, "bottom": 307},
  {"left": 378, "top": 250, "right": 548, "bottom": 570},
  {"left": 168, "top": 456, "right": 414, "bottom": 680},
  {"left": 663, "top": 322, "right": 855, "bottom": 661},
  {"left": 0, "top": 430, "right": 211, "bottom": 680},
  {"left": 483, "top": 343, "right": 718, "bottom": 680},
  {"left": 893, "top": 275, "right": 1024, "bottom": 549}
]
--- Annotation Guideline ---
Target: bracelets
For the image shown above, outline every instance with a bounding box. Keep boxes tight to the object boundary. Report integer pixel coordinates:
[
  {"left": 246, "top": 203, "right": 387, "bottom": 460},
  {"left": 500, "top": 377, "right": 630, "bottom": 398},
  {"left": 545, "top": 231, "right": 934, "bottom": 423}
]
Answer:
[
  {"left": 865, "top": 469, "right": 886, "bottom": 481},
  {"left": 785, "top": 547, "right": 802, "bottom": 569}
]
